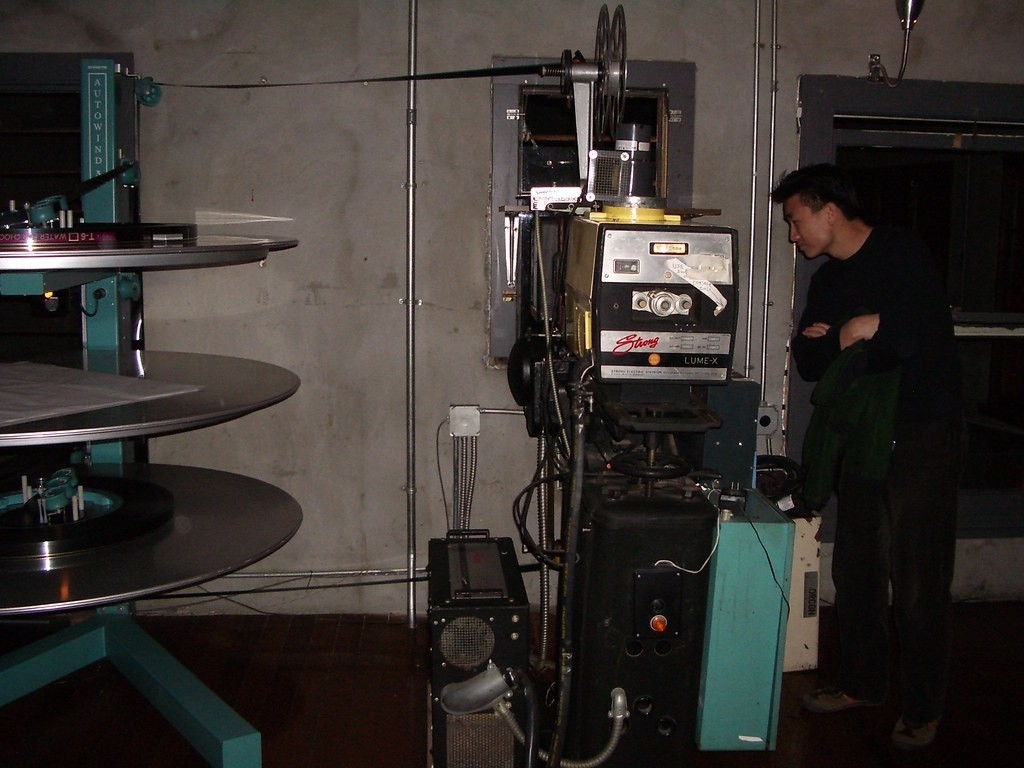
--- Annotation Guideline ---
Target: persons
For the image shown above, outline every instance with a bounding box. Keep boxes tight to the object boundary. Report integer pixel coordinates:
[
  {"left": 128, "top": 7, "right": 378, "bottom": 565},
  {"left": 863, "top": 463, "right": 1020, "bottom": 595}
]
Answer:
[{"left": 771, "top": 163, "right": 973, "bottom": 752}]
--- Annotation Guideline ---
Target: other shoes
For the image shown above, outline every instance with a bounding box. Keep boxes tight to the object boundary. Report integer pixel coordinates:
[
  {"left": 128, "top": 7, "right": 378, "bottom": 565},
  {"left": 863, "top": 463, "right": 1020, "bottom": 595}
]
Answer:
[
  {"left": 892, "top": 711, "right": 944, "bottom": 750},
  {"left": 800, "top": 686, "right": 880, "bottom": 713}
]
